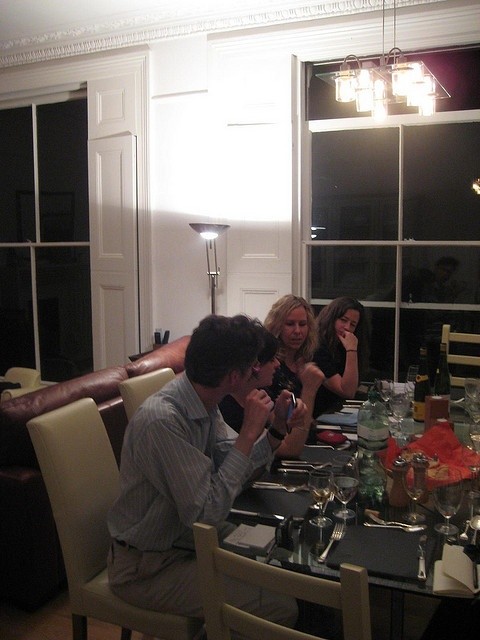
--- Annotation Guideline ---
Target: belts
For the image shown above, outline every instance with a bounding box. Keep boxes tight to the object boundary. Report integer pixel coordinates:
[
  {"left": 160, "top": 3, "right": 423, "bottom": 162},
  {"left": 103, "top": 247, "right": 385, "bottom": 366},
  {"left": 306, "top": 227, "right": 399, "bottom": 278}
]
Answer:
[{"left": 110, "top": 536, "right": 139, "bottom": 552}]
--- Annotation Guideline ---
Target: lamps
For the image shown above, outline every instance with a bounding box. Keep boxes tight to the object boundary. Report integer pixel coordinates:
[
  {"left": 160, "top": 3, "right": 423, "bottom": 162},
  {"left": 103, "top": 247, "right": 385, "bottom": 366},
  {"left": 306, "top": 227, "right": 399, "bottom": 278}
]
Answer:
[
  {"left": 189, "top": 223, "right": 232, "bottom": 316},
  {"left": 315, "top": 0, "right": 451, "bottom": 124}
]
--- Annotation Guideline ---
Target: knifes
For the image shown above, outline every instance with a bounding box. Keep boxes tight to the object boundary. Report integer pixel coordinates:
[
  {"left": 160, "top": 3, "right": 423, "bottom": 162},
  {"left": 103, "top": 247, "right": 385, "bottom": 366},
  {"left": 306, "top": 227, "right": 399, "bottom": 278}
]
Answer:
[
  {"left": 279, "top": 468, "right": 335, "bottom": 477},
  {"left": 417, "top": 534, "right": 428, "bottom": 581}
]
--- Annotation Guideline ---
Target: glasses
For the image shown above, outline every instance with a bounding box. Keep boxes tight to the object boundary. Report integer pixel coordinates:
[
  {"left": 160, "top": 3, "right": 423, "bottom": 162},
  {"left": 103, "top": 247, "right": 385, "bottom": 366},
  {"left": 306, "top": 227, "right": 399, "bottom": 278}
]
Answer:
[{"left": 245, "top": 359, "right": 261, "bottom": 375}]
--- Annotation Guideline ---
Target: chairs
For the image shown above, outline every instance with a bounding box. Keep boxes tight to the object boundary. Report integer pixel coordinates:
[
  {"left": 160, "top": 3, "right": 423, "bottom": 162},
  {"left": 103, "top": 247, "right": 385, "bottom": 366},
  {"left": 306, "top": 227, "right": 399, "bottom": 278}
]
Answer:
[
  {"left": 28, "top": 396, "right": 206, "bottom": 640},
  {"left": 434, "top": 325, "right": 480, "bottom": 390},
  {"left": 194, "top": 524, "right": 371, "bottom": 640},
  {"left": 119, "top": 367, "right": 176, "bottom": 423}
]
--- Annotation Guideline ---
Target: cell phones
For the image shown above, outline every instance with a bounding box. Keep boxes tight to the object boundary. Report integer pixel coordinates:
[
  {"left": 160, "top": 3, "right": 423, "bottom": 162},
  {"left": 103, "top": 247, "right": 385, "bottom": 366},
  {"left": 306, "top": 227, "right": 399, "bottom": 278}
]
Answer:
[{"left": 285, "top": 393, "right": 297, "bottom": 433}]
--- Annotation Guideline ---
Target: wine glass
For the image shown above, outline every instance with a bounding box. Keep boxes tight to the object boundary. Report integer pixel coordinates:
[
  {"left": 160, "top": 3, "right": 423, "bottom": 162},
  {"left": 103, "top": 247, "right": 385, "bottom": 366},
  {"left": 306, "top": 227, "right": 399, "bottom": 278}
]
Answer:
[
  {"left": 331, "top": 452, "right": 356, "bottom": 476},
  {"left": 432, "top": 466, "right": 461, "bottom": 535},
  {"left": 401, "top": 462, "right": 427, "bottom": 525},
  {"left": 333, "top": 476, "right": 357, "bottom": 519},
  {"left": 308, "top": 468, "right": 334, "bottom": 528},
  {"left": 376, "top": 379, "right": 394, "bottom": 414},
  {"left": 462, "top": 434, "right": 479, "bottom": 500},
  {"left": 405, "top": 365, "right": 421, "bottom": 401},
  {"left": 464, "top": 376, "right": 480, "bottom": 407},
  {"left": 464, "top": 398, "right": 480, "bottom": 433},
  {"left": 390, "top": 386, "right": 412, "bottom": 438}
]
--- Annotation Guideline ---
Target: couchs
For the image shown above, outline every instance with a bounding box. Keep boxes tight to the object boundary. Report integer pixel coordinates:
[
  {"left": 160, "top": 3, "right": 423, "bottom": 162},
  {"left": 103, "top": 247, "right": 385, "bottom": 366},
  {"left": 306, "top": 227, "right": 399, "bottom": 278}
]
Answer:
[
  {"left": 1, "top": 335, "right": 193, "bottom": 614},
  {"left": 1, "top": 368, "right": 45, "bottom": 402}
]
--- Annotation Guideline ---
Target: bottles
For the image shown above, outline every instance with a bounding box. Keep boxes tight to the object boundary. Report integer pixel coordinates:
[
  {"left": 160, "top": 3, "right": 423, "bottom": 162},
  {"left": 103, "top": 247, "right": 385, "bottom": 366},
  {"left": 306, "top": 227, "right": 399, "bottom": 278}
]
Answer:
[
  {"left": 409, "top": 453, "right": 428, "bottom": 504},
  {"left": 357, "top": 390, "right": 390, "bottom": 495},
  {"left": 413, "top": 347, "right": 430, "bottom": 422},
  {"left": 434, "top": 342, "right": 451, "bottom": 417},
  {"left": 388, "top": 454, "right": 409, "bottom": 513}
]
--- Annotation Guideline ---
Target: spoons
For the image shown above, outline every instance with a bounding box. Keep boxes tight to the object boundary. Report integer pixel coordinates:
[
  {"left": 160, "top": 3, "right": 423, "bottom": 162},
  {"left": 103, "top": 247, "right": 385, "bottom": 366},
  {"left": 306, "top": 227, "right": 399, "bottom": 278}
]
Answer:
[
  {"left": 469, "top": 513, "right": 479, "bottom": 546},
  {"left": 303, "top": 442, "right": 351, "bottom": 451},
  {"left": 364, "top": 522, "right": 430, "bottom": 533}
]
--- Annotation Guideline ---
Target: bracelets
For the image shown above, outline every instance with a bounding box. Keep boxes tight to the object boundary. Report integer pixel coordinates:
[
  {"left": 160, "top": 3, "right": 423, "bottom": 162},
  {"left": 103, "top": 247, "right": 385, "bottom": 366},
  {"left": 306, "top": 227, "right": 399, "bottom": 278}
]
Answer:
[
  {"left": 345, "top": 349, "right": 358, "bottom": 352},
  {"left": 295, "top": 424, "right": 310, "bottom": 432}
]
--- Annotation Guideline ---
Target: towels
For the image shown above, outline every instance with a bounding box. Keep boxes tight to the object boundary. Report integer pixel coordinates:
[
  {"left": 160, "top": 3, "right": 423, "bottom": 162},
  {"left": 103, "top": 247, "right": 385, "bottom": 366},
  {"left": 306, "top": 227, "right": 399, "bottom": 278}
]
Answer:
[
  {"left": 289, "top": 445, "right": 356, "bottom": 464},
  {"left": 314, "top": 413, "right": 357, "bottom": 425},
  {"left": 231, "top": 479, "right": 319, "bottom": 520},
  {"left": 376, "top": 419, "right": 479, "bottom": 497}
]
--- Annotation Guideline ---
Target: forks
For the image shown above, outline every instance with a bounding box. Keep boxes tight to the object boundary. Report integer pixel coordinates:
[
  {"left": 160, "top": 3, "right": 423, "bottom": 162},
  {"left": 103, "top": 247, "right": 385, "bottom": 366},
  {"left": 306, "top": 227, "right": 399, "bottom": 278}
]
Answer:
[
  {"left": 281, "top": 460, "right": 335, "bottom": 469},
  {"left": 460, "top": 519, "right": 471, "bottom": 541},
  {"left": 316, "top": 523, "right": 346, "bottom": 564},
  {"left": 252, "top": 482, "right": 305, "bottom": 493},
  {"left": 366, "top": 514, "right": 428, "bottom": 528}
]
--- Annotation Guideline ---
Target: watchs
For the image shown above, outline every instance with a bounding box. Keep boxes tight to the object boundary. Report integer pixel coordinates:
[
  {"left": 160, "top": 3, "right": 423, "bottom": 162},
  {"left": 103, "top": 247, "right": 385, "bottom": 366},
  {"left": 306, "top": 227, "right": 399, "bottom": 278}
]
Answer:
[{"left": 268, "top": 428, "right": 284, "bottom": 441}]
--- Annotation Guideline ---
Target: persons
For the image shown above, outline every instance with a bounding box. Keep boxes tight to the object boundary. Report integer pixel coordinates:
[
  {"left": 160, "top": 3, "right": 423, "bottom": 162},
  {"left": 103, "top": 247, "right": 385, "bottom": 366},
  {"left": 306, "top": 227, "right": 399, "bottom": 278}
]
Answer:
[
  {"left": 220, "top": 318, "right": 328, "bottom": 483},
  {"left": 312, "top": 297, "right": 370, "bottom": 420},
  {"left": 263, "top": 294, "right": 320, "bottom": 413},
  {"left": 107, "top": 315, "right": 299, "bottom": 638}
]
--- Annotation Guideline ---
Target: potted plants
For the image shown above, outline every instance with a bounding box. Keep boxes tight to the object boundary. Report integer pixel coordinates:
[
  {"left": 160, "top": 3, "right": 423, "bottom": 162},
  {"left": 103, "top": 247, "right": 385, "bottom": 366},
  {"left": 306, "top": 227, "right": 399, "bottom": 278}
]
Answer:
[{"left": 153, "top": 331, "right": 170, "bottom": 350}]
